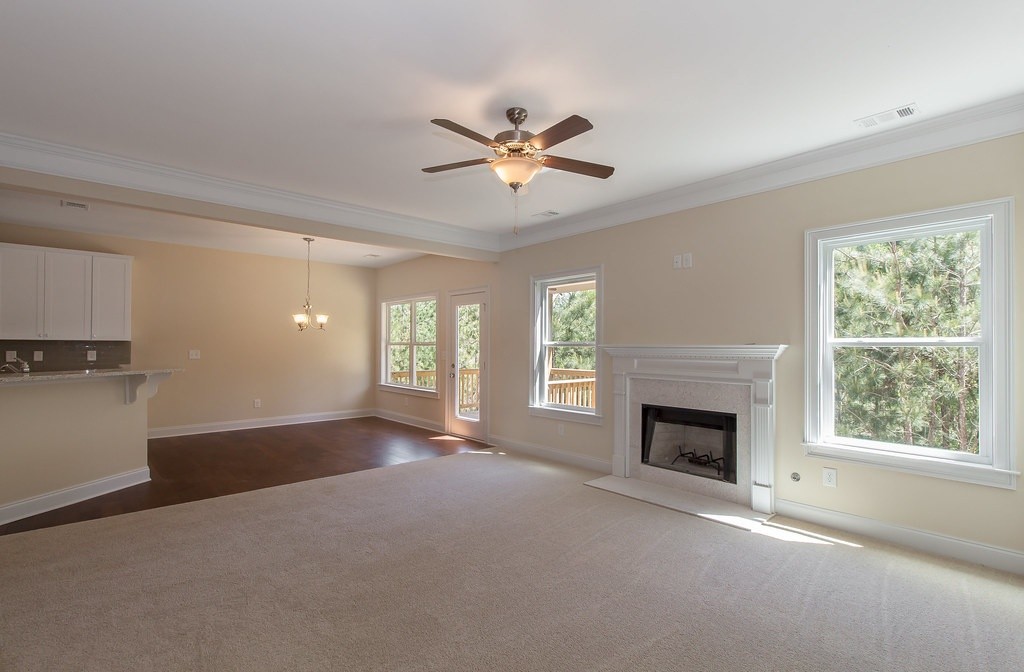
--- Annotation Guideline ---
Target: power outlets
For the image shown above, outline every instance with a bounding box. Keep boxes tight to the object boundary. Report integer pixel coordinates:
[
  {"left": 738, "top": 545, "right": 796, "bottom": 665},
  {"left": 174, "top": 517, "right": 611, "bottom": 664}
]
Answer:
[
  {"left": 6, "top": 351, "right": 17, "bottom": 363},
  {"left": 189, "top": 349, "right": 201, "bottom": 361},
  {"left": 33, "top": 350, "right": 44, "bottom": 362},
  {"left": 87, "top": 350, "right": 97, "bottom": 361},
  {"left": 821, "top": 467, "right": 836, "bottom": 488}
]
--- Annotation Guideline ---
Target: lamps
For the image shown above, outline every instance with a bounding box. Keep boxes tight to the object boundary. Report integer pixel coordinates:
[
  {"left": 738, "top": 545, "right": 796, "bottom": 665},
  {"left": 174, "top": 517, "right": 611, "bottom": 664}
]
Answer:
[{"left": 291, "top": 238, "right": 329, "bottom": 333}]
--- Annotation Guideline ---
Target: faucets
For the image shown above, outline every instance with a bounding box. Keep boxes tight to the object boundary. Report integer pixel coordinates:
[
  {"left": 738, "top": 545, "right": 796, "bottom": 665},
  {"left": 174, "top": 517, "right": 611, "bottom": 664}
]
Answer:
[{"left": 0, "top": 356, "right": 32, "bottom": 374}]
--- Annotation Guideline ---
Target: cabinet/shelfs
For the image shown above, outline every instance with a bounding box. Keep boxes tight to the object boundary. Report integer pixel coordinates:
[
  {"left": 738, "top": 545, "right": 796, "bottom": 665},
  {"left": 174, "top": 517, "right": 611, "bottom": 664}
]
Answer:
[{"left": 0, "top": 241, "right": 132, "bottom": 342}]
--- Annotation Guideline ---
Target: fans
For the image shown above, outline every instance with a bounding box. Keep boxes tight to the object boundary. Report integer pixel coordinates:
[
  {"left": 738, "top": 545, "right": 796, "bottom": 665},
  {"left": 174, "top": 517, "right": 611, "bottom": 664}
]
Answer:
[{"left": 420, "top": 106, "right": 618, "bottom": 195}]
